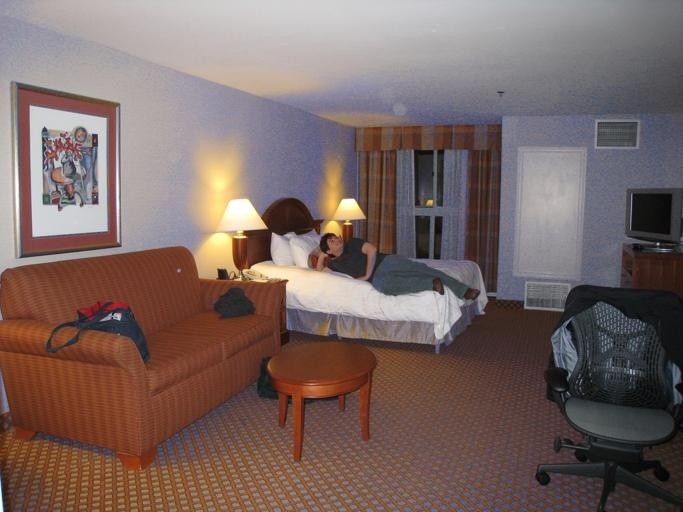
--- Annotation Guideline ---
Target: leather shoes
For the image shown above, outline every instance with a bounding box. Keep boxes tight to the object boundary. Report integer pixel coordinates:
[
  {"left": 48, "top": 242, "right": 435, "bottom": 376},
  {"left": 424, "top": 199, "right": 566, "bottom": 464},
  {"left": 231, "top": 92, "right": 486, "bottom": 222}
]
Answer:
[
  {"left": 464, "top": 288, "right": 480, "bottom": 300},
  {"left": 433, "top": 277, "right": 444, "bottom": 295}
]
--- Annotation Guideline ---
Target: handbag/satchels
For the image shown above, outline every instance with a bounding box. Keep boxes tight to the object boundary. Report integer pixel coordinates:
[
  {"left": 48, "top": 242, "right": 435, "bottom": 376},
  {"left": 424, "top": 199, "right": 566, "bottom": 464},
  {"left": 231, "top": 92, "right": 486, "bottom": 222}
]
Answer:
[{"left": 47, "top": 300, "right": 151, "bottom": 363}]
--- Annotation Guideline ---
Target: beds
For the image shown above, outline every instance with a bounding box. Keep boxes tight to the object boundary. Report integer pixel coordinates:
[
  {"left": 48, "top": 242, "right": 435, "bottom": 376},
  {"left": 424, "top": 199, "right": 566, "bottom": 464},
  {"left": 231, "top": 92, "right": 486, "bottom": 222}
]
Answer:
[{"left": 232, "top": 198, "right": 482, "bottom": 354}]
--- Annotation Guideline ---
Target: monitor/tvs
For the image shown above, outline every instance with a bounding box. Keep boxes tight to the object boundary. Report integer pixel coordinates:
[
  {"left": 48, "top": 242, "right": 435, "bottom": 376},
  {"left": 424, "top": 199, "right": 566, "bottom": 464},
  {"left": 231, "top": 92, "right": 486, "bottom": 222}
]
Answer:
[{"left": 624, "top": 187, "right": 683, "bottom": 251}]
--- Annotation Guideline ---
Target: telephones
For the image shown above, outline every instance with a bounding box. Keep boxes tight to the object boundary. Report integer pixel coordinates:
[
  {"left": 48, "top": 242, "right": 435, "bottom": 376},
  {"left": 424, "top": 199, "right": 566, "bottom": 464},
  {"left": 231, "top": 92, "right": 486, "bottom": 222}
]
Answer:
[{"left": 243, "top": 268, "right": 268, "bottom": 280}]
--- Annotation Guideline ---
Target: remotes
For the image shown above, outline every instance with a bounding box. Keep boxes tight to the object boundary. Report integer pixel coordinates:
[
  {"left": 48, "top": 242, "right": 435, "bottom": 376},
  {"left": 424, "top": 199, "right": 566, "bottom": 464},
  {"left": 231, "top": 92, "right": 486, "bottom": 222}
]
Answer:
[{"left": 631, "top": 243, "right": 643, "bottom": 250}]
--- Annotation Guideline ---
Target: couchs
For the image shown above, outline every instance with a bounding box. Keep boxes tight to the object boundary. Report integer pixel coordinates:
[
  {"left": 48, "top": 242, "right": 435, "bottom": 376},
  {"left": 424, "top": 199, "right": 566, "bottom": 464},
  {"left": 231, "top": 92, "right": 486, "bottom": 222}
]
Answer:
[{"left": 0, "top": 246, "right": 286, "bottom": 473}]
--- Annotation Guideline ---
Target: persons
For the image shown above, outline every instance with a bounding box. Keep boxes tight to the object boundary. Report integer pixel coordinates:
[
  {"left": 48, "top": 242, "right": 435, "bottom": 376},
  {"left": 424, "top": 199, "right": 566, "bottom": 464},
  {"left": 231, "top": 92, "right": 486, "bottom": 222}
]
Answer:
[{"left": 316, "top": 232, "right": 481, "bottom": 301}]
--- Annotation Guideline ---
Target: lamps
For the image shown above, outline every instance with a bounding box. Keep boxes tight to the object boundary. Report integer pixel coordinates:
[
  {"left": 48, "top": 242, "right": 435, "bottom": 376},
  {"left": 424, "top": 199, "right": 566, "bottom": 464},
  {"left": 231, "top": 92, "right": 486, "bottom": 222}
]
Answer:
[
  {"left": 331, "top": 198, "right": 367, "bottom": 243},
  {"left": 216, "top": 198, "right": 268, "bottom": 272}
]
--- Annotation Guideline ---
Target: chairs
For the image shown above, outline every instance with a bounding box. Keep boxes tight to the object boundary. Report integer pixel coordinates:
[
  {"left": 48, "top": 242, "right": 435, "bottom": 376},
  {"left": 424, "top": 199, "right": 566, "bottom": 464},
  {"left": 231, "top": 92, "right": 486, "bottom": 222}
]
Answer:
[{"left": 535, "top": 285, "right": 683, "bottom": 510}]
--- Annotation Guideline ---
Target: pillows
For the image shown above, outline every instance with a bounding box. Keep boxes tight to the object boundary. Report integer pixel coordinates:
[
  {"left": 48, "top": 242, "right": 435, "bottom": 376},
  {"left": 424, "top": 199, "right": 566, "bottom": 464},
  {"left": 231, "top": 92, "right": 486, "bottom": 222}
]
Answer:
[{"left": 270, "top": 229, "right": 333, "bottom": 271}]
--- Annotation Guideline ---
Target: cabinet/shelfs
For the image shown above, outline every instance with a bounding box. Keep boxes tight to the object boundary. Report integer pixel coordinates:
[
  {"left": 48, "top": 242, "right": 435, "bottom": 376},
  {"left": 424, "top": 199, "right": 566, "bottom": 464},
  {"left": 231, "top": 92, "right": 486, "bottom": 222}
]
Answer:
[{"left": 620, "top": 243, "right": 683, "bottom": 296}]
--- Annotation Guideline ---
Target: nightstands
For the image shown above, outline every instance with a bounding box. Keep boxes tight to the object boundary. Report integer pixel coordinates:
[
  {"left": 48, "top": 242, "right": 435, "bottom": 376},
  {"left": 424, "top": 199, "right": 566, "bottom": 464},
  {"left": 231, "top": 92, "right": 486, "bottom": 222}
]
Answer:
[{"left": 267, "top": 279, "right": 289, "bottom": 347}]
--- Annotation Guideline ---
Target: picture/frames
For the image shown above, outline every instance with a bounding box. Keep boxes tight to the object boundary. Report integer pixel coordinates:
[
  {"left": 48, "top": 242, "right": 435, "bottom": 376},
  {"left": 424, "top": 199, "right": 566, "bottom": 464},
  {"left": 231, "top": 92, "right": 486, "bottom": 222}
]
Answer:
[{"left": 12, "top": 81, "right": 122, "bottom": 258}]
